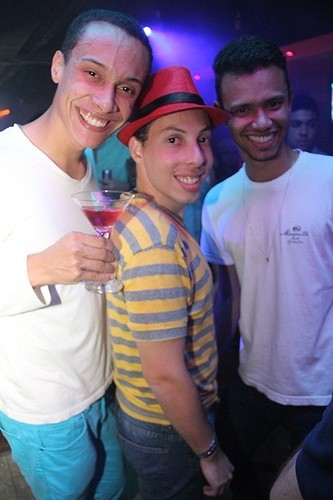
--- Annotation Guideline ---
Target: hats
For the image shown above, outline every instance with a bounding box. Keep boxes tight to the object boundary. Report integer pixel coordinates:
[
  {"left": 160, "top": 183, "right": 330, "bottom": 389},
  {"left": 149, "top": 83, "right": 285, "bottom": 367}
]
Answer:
[{"left": 116, "top": 66, "right": 224, "bottom": 147}]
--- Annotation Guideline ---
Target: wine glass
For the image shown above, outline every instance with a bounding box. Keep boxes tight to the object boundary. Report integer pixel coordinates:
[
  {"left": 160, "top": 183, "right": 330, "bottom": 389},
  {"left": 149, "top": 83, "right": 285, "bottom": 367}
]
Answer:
[{"left": 73, "top": 191, "right": 135, "bottom": 294}]
